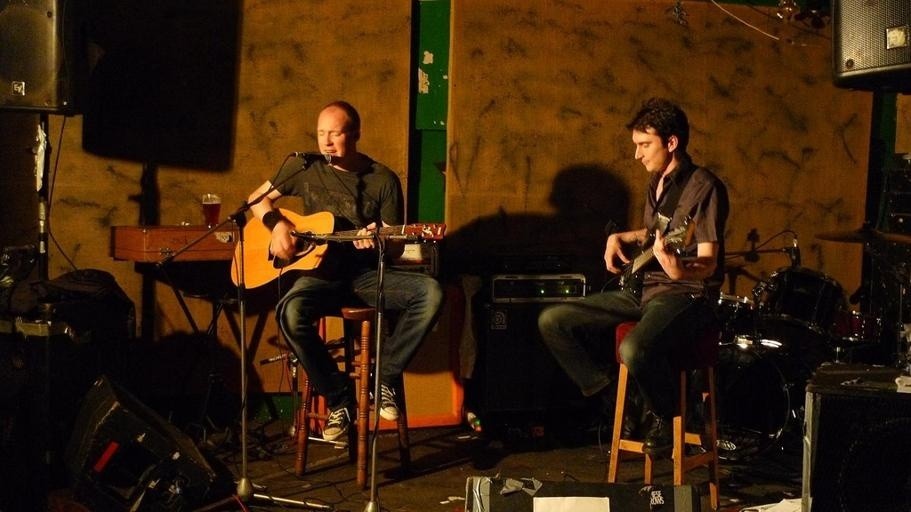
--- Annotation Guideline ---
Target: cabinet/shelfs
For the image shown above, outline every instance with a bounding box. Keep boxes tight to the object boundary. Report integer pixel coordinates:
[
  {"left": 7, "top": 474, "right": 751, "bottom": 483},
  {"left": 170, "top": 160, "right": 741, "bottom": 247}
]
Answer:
[{"left": 799, "top": 359, "right": 910, "bottom": 512}]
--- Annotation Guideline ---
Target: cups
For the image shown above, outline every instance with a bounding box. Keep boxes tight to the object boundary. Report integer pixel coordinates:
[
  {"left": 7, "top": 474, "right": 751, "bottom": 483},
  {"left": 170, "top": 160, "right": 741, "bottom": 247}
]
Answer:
[{"left": 201, "top": 191, "right": 221, "bottom": 226}]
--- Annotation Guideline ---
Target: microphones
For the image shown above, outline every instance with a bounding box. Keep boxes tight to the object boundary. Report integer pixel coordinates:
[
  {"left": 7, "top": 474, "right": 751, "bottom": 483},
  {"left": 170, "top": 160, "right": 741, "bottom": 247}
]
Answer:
[
  {"left": 790, "top": 237, "right": 800, "bottom": 266},
  {"left": 290, "top": 231, "right": 325, "bottom": 245},
  {"left": 294, "top": 152, "right": 333, "bottom": 163}
]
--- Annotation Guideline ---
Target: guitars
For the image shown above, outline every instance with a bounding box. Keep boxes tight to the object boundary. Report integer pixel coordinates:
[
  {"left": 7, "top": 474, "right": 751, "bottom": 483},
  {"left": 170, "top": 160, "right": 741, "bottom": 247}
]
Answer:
[
  {"left": 230, "top": 208, "right": 448, "bottom": 288},
  {"left": 619, "top": 216, "right": 694, "bottom": 290}
]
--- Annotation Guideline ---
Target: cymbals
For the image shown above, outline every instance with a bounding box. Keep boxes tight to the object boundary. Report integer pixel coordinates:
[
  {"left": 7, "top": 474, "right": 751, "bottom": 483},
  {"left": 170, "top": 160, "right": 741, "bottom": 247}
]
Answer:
[{"left": 814, "top": 226, "right": 911, "bottom": 244}]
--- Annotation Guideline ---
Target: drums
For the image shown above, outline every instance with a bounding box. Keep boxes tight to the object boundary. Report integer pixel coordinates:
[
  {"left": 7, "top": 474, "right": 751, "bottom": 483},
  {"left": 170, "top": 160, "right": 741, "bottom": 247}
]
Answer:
[
  {"left": 699, "top": 335, "right": 803, "bottom": 462},
  {"left": 719, "top": 294, "right": 755, "bottom": 345},
  {"left": 756, "top": 268, "right": 842, "bottom": 343},
  {"left": 840, "top": 311, "right": 882, "bottom": 348}
]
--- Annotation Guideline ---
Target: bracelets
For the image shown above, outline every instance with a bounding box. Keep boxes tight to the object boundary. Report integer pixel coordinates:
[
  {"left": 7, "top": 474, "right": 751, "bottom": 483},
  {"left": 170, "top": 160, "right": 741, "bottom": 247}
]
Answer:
[{"left": 262, "top": 211, "right": 282, "bottom": 231}]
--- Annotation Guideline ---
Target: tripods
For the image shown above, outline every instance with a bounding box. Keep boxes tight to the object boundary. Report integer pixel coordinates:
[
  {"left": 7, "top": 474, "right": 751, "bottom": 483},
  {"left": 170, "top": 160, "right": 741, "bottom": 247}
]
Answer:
[{"left": 197, "top": 216, "right": 330, "bottom": 512}]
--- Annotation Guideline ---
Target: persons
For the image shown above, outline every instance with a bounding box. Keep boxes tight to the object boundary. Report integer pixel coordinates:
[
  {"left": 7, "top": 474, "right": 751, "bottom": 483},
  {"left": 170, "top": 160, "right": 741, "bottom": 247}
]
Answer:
[
  {"left": 246, "top": 98, "right": 441, "bottom": 442},
  {"left": 535, "top": 93, "right": 730, "bottom": 456}
]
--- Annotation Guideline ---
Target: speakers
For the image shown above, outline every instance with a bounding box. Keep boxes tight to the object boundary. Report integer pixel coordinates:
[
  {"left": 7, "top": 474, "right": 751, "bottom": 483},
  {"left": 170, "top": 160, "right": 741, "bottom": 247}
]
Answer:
[
  {"left": 465, "top": 477, "right": 701, "bottom": 512},
  {"left": 0, "top": 0, "right": 78, "bottom": 111},
  {"left": 832, "top": 0, "right": 911, "bottom": 90}
]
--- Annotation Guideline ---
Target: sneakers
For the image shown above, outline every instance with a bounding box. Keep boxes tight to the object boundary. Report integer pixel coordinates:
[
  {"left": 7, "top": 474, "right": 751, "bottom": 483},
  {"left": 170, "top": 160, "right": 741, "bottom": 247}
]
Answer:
[
  {"left": 322, "top": 404, "right": 351, "bottom": 439},
  {"left": 372, "top": 383, "right": 399, "bottom": 421}
]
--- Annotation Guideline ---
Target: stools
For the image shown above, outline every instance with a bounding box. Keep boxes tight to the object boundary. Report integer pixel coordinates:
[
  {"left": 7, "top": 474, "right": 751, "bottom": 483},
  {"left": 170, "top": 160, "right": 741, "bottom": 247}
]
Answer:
[
  {"left": 608, "top": 321, "right": 720, "bottom": 512},
  {"left": 293, "top": 302, "right": 415, "bottom": 492}
]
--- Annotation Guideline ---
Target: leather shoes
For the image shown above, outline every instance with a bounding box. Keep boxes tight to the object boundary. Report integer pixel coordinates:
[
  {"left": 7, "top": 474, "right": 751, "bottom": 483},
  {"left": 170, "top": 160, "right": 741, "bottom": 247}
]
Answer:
[
  {"left": 613, "top": 397, "right": 640, "bottom": 440},
  {"left": 644, "top": 418, "right": 681, "bottom": 453}
]
